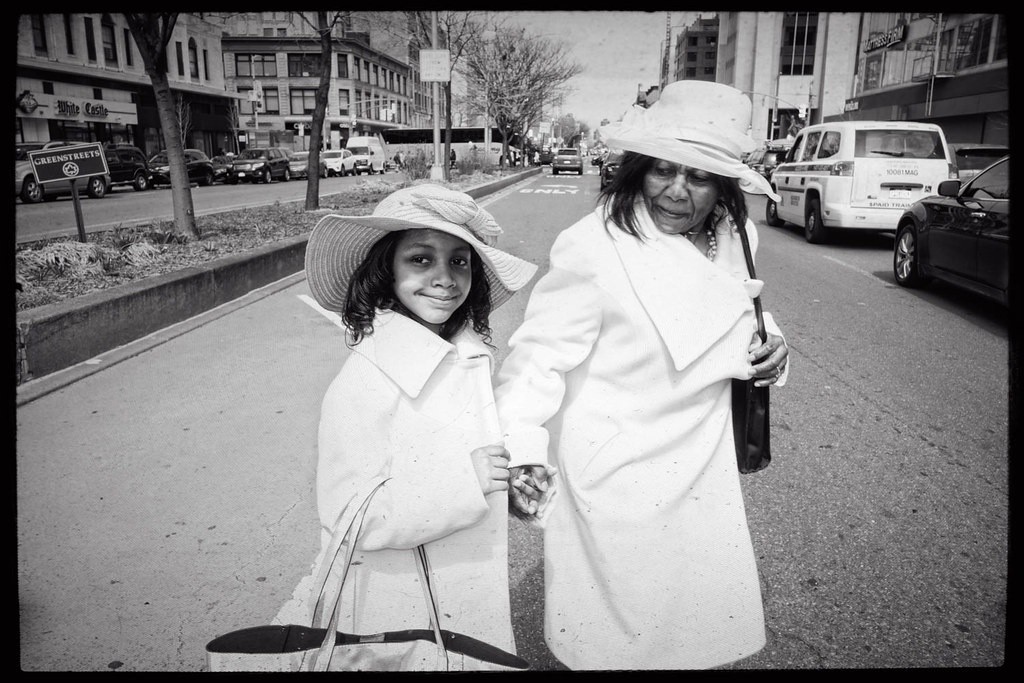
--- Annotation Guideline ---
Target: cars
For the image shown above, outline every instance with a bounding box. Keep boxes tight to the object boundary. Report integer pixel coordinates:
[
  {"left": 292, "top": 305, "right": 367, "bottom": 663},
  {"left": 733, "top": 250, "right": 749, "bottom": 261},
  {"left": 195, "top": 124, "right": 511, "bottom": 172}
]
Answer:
[
  {"left": 286, "top": 150, "right": 327, "bottom": 179},
  {"left": 148, "top": 149, "right": 213, "bottom": 189},
  {"left": 892, "top": 152, "right": 1011, "bottom": 308},
  {"left": 591, "top": 150, "right": 622, "bottom": 191},
  {"left": 209, "top": 156, "right": 239, "bottom": 184}
]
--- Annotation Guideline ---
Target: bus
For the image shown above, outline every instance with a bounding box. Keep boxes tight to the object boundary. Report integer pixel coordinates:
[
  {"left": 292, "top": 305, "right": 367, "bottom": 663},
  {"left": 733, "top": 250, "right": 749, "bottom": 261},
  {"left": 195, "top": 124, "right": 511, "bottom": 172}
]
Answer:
[{"left": 380, "top": 130, "right": 523, "bottom": 167}]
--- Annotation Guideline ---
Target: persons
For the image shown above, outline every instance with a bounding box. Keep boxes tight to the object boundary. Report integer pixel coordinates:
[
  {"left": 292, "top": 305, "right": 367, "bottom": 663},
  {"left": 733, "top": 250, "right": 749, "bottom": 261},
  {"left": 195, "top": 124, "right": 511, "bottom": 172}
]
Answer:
[
  {"left": 533, "top": 150, "right": 541, "bottom": 167},
  {"left": 494, "top": 79, "right": 789, "bottom": 670},
  {"left": 394, "top": 149, "right": 456, "bottom": 172},
  {"left": 267, "top": 184, "right": 557, "bottom": 653}
]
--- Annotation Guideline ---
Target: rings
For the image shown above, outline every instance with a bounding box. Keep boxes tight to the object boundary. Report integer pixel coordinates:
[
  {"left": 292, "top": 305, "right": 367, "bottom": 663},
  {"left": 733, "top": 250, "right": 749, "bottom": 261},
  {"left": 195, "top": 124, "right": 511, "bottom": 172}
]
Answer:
[{"left": 775, "top": 366, "right": 780, "bottom": 378}]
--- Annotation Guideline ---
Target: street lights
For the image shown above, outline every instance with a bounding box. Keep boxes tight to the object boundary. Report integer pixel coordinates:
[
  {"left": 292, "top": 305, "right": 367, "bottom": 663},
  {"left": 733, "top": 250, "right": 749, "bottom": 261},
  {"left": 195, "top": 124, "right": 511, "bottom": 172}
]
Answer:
[{"left": 250, "top": 53, "right": 263, "bottom": 149}]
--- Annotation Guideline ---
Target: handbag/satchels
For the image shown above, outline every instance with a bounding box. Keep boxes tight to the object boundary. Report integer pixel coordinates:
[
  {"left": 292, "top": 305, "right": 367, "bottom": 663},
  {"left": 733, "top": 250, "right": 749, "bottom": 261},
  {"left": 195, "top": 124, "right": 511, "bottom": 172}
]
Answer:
[
  {"left": 726, "top": 201, "right": 772, "bottom": 473},
  {"left": 207, "top": 478, "right": 531, "bottom": 672}
]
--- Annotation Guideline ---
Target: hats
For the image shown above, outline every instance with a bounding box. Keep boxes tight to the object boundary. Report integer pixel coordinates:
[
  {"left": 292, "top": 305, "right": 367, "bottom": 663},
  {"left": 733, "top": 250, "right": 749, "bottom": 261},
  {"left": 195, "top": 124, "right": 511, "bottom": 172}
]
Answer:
[
  {"left": 304, "top": 184, "right": 539, "bottom": 320},
  {"left": 599, "top": 79, "right": 782, "bottom": 202}
]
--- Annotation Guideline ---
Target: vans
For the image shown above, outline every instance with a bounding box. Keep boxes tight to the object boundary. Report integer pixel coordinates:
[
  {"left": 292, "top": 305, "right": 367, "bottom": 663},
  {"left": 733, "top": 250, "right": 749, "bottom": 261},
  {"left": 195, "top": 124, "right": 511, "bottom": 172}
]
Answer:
[
  {"left": 345, "top": 137, "right": 386, "bottom": 175},
  {"left": 766, "top": 120, "right": 961, "bottom": 243}
]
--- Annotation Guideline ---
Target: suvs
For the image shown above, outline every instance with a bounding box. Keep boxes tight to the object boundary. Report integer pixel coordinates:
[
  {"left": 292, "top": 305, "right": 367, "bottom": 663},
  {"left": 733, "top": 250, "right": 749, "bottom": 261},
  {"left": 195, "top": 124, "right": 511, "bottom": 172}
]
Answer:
[
  {"left": 101, "top": 141, "right": 152, "bottom": 192},
  {"left": 234, "top": 147, "right": 294, "bottom": 183},
  {"left": 15, "top": 140, "right": 111, "bottom": 203},
  {"left": 321, "top": 148, "right": 357, "bottom": 177},
  {"left": 552, "top": 148, "right": 584, "bottom": 175},
  {"left": 744, "top": 143, "right": 794, "bottom": 185},
  {"left": 528, "top": 146, "right": 552, "bottom": 166}
]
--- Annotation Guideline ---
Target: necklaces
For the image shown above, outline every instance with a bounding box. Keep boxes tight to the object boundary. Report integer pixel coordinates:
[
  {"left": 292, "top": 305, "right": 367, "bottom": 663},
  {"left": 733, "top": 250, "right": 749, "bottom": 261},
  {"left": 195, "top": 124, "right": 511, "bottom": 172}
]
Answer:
[{"left": 706, "top": 230, "right": 716, "bottom": 260}]
